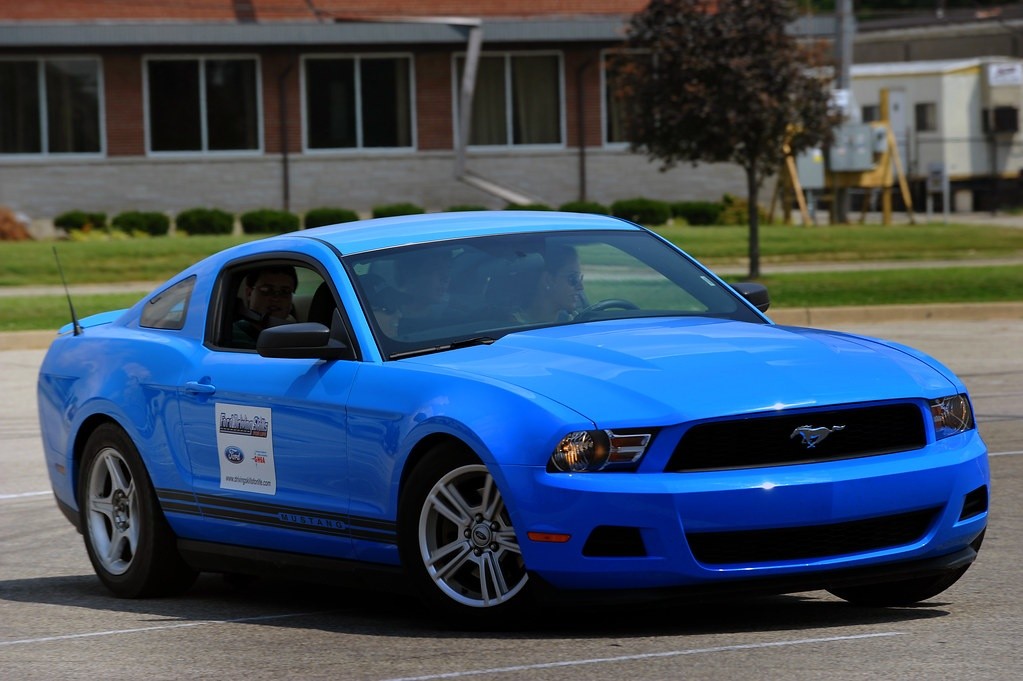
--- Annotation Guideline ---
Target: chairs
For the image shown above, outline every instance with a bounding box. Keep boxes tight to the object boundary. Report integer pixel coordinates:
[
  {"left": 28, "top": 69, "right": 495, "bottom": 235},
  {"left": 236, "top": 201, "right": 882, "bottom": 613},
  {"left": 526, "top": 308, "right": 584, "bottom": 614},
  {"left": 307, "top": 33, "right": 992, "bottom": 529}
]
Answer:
[
  {"left": 308, "top": 274, "right": 382, "bottom": 334},
  {"left": 467, "top": 252, "right": 547, "bottom": 323}
]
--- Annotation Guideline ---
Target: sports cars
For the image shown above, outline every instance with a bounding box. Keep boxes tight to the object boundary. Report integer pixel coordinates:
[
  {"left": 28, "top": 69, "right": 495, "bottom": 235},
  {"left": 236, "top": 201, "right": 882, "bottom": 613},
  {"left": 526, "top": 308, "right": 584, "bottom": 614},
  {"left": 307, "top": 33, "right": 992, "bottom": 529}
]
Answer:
[{"left": 37, "top": 210, "right": 990, "bottom": 628}]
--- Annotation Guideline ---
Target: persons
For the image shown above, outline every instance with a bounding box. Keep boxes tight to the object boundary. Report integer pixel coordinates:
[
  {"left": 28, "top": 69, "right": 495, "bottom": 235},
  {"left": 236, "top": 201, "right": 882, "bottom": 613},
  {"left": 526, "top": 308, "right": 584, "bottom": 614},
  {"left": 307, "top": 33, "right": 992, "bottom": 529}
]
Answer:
[
  {"left": 231, "top": 266, "right": 299, "bottom": 347},
  {"left": 357, "top": 252, "right": 454, "bottom": 339},
  {"left": 508, "top": 246, "right": 584, "bottom": 325}
]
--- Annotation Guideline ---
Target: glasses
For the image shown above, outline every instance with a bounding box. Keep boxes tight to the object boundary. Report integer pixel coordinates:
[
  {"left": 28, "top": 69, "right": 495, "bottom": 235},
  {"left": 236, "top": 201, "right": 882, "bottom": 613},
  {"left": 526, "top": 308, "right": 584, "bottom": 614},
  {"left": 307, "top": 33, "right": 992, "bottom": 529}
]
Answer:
[
  {"left": 370, "top": 302, "right": 398, "bottom": 315},
  {"left": 558, "top": 270, "right": 584, "bottom": 286}
]
